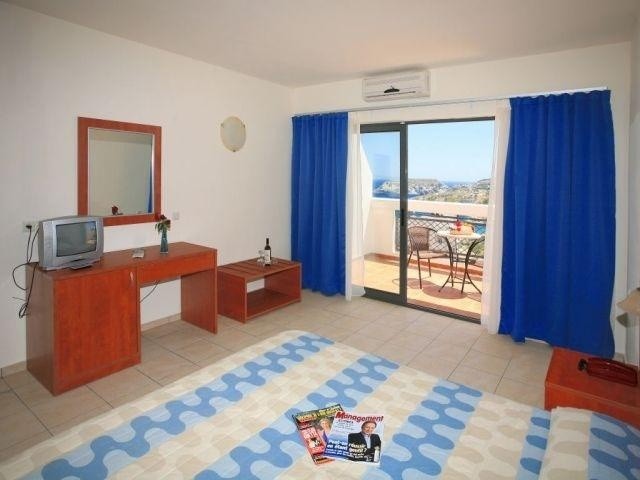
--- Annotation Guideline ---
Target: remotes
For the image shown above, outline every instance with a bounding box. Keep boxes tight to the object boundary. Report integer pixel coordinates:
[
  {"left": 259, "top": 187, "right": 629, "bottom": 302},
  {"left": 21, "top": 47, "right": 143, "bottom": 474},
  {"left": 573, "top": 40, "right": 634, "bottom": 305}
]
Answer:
[{"left": 132, "top": 247, "right": 144, "bottom": 258}]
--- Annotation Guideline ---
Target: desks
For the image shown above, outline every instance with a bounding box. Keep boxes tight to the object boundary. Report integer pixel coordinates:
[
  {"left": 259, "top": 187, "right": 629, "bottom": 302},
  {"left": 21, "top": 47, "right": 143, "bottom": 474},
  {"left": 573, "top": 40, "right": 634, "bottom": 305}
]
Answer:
[
  {"left": 21, "top": 242, "right": 217, "bottom": 395},
  {"left": 437, "top": 229, "right": 486, "bottom": 295}
]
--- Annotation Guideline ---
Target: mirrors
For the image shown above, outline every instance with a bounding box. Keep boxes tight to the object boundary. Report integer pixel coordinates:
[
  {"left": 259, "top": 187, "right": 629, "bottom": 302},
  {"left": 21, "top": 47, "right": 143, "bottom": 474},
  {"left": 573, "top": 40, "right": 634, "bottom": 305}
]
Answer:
[{"left": 77, "top": 117, "right": 162, "bottom": 226}]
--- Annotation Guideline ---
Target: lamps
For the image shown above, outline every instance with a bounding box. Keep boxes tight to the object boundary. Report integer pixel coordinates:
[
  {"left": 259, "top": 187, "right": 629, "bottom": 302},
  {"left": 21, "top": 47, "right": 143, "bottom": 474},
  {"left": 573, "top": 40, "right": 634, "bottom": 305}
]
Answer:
[{"left": 617, "top": 287, "right": 640, "bottom": 319}]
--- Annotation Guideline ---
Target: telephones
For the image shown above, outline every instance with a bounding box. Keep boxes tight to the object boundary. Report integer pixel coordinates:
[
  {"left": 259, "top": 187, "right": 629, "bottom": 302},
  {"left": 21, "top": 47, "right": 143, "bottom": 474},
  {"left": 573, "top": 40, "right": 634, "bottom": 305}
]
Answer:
[{"left": 578, "top": 356, "right": 639, "bottom": 386}]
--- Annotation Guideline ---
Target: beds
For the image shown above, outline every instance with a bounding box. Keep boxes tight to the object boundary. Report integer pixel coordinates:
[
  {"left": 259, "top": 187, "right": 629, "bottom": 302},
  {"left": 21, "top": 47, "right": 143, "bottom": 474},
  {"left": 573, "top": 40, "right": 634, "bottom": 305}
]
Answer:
[{"left": 0, "top": 329, "right": 640, "bottom": 479}]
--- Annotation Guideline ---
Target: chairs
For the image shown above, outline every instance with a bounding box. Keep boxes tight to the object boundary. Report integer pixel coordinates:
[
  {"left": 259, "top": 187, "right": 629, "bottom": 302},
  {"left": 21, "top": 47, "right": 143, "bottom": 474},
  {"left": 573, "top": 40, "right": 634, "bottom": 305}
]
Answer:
[{"left": 408, "top": 226, "right": 454, "bottom": 289}]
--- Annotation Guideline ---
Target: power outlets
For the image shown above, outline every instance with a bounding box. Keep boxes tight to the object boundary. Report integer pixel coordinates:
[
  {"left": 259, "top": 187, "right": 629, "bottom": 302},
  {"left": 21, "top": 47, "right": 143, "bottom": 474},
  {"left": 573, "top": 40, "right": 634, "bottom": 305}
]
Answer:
[{"left": 23, "top": 222, "right": 35, "bottom": 232}]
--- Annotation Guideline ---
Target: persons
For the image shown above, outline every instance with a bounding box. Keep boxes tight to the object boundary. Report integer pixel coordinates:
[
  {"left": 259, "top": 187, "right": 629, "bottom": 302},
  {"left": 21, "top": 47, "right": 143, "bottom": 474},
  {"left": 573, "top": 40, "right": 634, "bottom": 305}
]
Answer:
[
  {"left": 316, "top": 417, "right": 333, "bottom": 444},
  {"left": 347, "top": 421, "right": 381, "bottom": 462}
]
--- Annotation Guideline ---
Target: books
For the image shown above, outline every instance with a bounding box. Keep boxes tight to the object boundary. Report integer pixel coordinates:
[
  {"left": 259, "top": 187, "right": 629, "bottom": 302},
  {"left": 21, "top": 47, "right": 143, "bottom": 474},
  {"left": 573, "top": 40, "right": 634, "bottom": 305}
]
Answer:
[
  {"left": 292, "top": 403, "right": 344, "bottom": 465},
  {"left": 321, "top": 410, "right": 385, "bottom": 468}
]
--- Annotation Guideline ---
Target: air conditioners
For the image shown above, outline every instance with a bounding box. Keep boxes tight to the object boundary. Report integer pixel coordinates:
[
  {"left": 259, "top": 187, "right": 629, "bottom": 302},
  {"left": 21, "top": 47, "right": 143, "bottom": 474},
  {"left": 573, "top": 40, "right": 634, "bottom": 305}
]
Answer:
[{"left": 360, "top": 69, "right": 431, "bottom": 100}]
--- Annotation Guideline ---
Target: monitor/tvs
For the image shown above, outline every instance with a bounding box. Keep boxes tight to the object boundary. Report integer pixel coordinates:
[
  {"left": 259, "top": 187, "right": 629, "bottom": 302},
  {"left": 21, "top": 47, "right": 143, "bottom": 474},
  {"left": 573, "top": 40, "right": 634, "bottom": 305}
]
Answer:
[{"left": 38, "top": 216, "right": 104, "bottom": 270}]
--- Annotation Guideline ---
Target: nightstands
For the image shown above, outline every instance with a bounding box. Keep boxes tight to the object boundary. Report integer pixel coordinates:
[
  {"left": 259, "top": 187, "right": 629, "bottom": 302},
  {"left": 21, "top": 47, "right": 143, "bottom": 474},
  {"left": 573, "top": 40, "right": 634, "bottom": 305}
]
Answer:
[{"left": 545, "top": 346, "right": 640, "bottom": 431}]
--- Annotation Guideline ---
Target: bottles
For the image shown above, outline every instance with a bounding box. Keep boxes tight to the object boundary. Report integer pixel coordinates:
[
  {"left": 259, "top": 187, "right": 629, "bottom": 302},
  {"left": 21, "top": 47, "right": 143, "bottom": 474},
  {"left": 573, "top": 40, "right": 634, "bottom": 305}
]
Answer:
[
  {"left": 264, "top": 237, "right": 272, "bottom": 267},
  {"left": 456, "top": 215, "right": 461, "bottom": 232}
]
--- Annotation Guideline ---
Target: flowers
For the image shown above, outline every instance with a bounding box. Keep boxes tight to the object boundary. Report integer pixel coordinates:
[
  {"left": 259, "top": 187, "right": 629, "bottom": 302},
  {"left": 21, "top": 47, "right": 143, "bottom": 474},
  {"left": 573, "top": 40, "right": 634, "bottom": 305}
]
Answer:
[{"left": 155, "top": 213, "right": 171, "bottom": 232}]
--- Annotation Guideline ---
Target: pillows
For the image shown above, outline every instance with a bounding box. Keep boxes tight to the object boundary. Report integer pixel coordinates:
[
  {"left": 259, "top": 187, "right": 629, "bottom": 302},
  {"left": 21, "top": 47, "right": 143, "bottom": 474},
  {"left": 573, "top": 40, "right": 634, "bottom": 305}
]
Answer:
[{"left": 538, "top": 405, "right": 640, "bottom": 480}]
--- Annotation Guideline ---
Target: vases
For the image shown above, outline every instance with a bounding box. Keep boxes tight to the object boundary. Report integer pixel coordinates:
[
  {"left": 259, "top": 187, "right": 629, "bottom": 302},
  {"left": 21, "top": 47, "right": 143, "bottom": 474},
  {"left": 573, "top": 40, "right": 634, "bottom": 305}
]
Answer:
[{"left": 159, "top": 232, "right": 169, "bottom": 253}]
